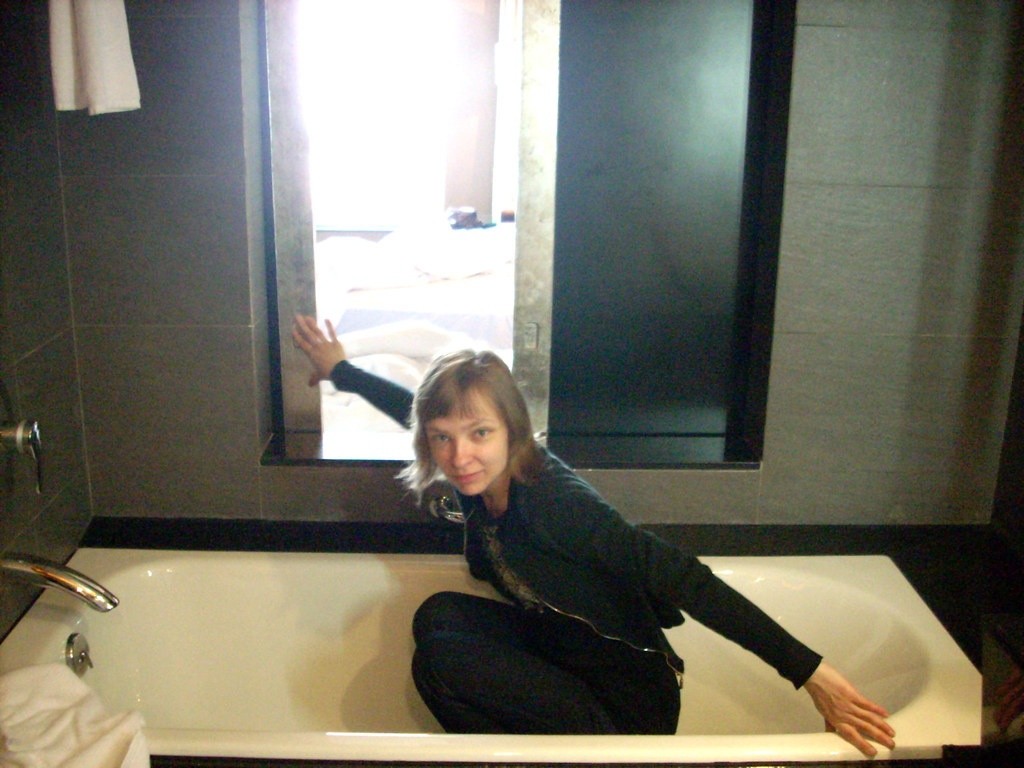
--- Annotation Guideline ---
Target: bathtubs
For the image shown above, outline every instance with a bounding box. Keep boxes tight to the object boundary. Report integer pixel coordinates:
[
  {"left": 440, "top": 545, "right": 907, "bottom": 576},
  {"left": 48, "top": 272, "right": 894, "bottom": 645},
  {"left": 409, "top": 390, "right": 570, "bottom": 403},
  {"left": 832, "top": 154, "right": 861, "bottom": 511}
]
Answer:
[{"left": 1, "top": 546, "right": 983, "bottom": 764}]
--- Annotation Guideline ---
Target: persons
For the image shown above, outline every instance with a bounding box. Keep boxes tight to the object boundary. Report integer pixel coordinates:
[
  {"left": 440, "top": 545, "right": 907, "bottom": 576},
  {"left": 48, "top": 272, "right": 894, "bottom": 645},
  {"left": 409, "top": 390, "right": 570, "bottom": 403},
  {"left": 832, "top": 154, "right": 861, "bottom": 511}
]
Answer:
[{"left": 289, "top": 311, "right": 897, "bottom": 758}]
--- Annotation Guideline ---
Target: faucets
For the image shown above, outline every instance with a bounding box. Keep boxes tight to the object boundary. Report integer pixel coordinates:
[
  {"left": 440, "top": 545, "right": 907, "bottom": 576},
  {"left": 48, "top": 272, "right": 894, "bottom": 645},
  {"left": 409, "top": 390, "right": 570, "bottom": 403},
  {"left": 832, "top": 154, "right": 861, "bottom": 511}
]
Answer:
[{"left": 0, "top": 555, "right": 119, "bottom": 613}]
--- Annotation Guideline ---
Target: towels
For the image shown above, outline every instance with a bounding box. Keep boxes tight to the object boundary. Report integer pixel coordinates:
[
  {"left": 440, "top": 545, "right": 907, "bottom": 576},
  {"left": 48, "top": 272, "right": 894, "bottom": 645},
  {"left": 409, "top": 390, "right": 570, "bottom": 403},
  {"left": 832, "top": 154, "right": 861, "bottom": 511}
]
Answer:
[
  {"left": 0, "top": 663, "right": 150, "bottom": 768},
  {"left": 50, "top": 0, "right": 143, "bottom": 116}
]
